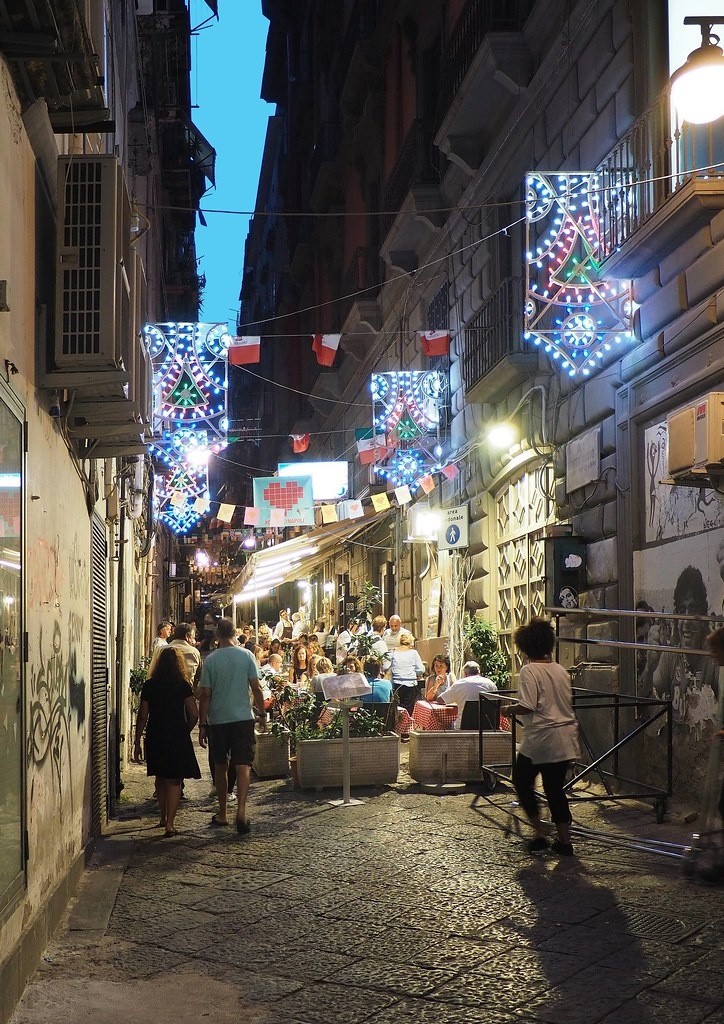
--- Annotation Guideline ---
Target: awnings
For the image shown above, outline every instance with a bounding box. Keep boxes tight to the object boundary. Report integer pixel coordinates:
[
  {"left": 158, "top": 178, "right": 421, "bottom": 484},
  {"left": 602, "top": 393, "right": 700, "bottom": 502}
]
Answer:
[{"left": 215, "top": 502, "right": 393, "bottom": 608}]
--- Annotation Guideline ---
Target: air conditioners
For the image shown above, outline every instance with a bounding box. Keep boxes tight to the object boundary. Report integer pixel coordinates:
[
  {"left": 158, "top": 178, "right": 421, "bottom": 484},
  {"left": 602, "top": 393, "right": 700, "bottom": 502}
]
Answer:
[
  {"left": 657, "top": 392, "right": 724, "bottom": 489},
  {"left": 39, "top": 155, "right": 155, "bottom": 460}
]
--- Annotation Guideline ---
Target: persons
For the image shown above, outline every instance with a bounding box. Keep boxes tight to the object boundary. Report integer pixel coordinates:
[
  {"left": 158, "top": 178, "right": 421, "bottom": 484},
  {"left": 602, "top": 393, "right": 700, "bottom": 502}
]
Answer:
[
  {"left": 636, "top": 565, "right": 724, "bottom": 733},
  {"left": 134, "top": 608, "right": 501, "bottom": 838},
  {"left": 500, "top": 620, "right": 581, "bottom": 856}
]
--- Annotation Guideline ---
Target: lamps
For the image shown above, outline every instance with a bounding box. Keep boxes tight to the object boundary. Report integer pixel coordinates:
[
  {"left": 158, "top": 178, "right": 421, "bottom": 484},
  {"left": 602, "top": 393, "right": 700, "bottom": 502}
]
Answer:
[{"left": 670, "top": 15, "right": 724, "bottom": 128}]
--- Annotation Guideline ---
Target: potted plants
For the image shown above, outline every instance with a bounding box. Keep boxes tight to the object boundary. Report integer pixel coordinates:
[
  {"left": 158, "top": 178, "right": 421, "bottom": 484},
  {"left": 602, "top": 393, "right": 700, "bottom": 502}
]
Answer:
[{"left": 259, "top": 581, "right": 400, "bottom": 793}]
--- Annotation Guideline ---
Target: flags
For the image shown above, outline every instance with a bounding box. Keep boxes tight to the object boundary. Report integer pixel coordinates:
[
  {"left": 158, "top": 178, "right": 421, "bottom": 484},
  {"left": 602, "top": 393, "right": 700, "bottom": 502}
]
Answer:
[
  {"left": 312, "top": 334, "right": 344, "bottom": 367},
  {"left": 171, "top": 429, "right": 460, "bottom": 529},
  {"left": 228, "top": 335, "right": 260, "bottom": 365},
  {"left": 421, "top": 330, "right": 449, "bottom": 356}
]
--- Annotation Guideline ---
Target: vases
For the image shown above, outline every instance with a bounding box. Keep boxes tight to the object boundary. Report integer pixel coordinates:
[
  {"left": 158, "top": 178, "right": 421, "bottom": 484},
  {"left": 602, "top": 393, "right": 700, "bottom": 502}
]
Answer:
[
  {"left": 249, "top": 719, "right": 290, "bottom": 777},
  {"left": 408, "top": 730, "right": 513, "bottom": 784}
]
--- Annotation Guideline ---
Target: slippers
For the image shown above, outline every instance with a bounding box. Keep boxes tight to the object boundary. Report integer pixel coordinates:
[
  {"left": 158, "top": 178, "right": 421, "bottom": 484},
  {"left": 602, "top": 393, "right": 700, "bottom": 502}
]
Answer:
[
  {"left": 236, "top": 819, "right": 251, "bottom": 833},
  {"left": 212, "top": 813, "right": 228, "bottom": 826},
  {"left": 160, "top": 819, "right": 166, "bottom": 826},
  {"left": 166, "top": 830, "right": 178, "bottom": 837}
]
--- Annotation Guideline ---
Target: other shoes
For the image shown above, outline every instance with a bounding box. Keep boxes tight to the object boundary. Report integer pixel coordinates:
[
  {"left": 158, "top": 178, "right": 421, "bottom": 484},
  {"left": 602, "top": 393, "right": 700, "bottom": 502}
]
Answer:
[
  {"left": 401, "top": 736, "right": 410, "bottom": 743},
  {"left": 153, "top": 790, "right": 159, "bottom": 797},
  {"left": 551, "top": 841, "right": 573, "bottom": 855},
  {"left": 226, "top": 792, "right": 236, "bottom": 802},
  {"left": 209, "top": 789, "right": 217, "bottom": 797},
  {"left": 527, "top": 838, "right": 547, "bottom": 850}
]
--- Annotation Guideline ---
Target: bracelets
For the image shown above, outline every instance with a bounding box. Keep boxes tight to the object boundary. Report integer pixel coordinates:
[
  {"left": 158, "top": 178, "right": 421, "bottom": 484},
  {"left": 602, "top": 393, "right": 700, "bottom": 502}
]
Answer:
[
  {"left": 199, "top": 722, "right": 208, "bottom": 728},
  {"left": 134, "top": 740, "right": 140, "bottom": 745}
]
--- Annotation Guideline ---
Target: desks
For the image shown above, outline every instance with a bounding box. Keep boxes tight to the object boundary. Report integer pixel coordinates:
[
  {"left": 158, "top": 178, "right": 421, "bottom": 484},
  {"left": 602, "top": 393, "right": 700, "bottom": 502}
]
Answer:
[
  {"left": 410, "top": 700, "right": 516, "bottom": 731},
  {"left": 318, "top": 705, "right": 413, "bottom": 739},
  {"left": 277, "top": 680, "right": 412, "bottom": 739}
]
